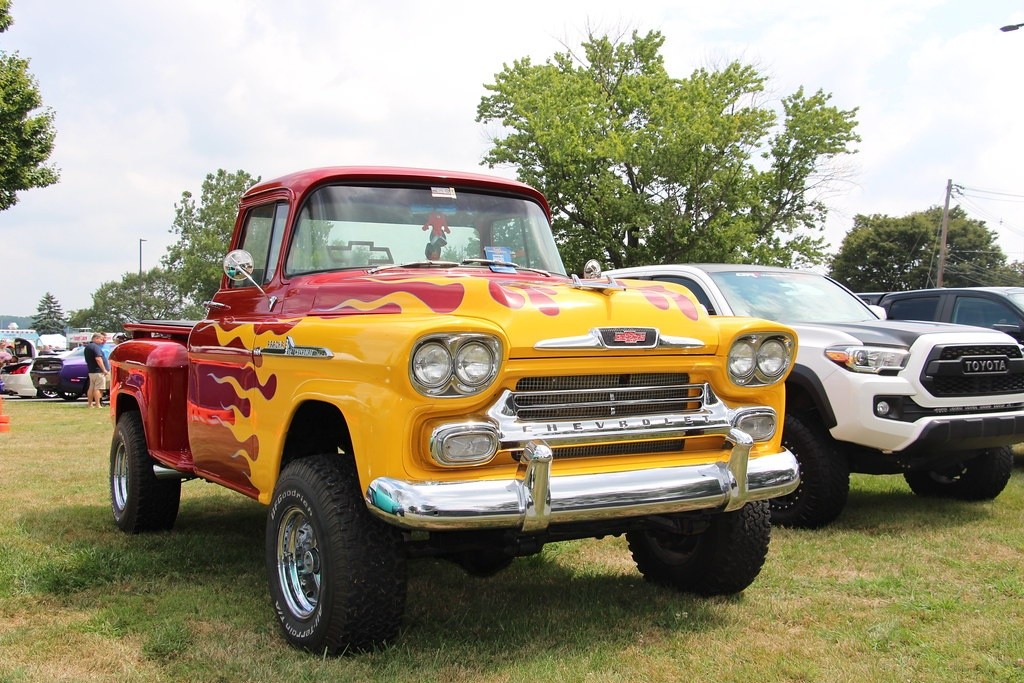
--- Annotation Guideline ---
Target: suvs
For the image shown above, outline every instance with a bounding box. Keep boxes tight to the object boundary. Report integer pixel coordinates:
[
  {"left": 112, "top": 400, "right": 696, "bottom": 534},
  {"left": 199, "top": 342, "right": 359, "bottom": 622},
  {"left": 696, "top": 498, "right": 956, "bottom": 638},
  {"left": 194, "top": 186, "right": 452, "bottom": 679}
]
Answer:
[
  {"left": 852, "top": 287, "right": 1024, "bottom": 346},
  {"left": 601, "top": 263, "right": 1024, "bottom": 529}
]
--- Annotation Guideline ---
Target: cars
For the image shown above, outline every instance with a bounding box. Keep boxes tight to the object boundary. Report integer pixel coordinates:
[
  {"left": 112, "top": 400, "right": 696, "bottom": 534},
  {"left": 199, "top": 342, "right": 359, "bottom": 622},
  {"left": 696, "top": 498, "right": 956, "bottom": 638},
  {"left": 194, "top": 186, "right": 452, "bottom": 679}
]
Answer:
[
  {"left": 31, "top": 344, "right": 116, "bottom": 401},
  {"left": 1, "top": 349, "right": 78, "bottom": 399}
]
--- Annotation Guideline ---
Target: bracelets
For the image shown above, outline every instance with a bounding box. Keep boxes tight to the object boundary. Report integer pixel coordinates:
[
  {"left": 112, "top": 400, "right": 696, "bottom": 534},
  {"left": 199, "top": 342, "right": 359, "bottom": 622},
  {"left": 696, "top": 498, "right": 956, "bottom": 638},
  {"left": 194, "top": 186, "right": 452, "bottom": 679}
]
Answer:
[{"left": 105, "top": 372, "right": 108, "bottom": 375}]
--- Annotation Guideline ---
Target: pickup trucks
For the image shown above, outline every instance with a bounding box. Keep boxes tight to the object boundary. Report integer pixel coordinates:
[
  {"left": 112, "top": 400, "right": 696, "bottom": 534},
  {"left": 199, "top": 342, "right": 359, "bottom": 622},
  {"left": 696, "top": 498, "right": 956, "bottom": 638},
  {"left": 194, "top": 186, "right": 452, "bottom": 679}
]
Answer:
[{"left": 109, "top": 166, "right": 800, "bottom": 655}]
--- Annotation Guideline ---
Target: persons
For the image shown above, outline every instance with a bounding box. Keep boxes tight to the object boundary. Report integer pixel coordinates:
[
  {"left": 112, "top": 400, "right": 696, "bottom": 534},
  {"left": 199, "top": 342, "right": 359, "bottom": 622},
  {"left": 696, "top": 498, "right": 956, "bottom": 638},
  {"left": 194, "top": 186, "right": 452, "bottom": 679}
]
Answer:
[
  {"left": 83, "top": 332, "right": 110, "bottom": 408},
  {"left": 38, "top": 345, "right": 60, "bottom": 356},
  {"left": 78, "top": 343, "right": 83, "bottom": 350},
  {"left": 0, "top": 339, "right": 18, "bottom": 366}
]
